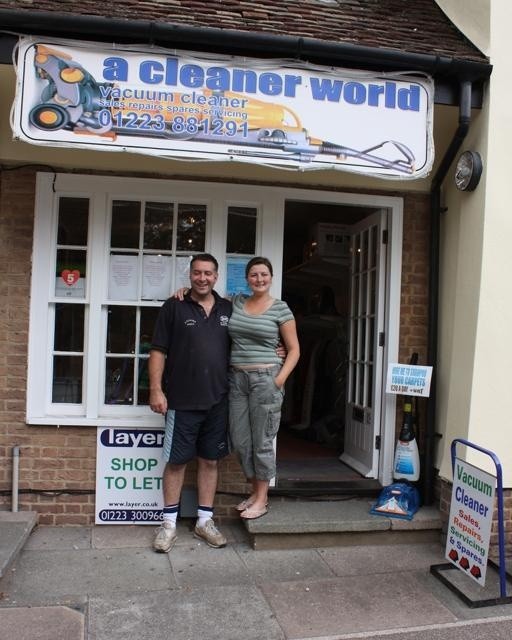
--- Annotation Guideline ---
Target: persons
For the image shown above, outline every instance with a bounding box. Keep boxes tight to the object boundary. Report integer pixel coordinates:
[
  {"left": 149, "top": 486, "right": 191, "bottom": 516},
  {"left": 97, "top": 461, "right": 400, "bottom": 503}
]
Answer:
[
  {"left": 150, "top": 253, "right": 286, "bottom": 553},
  {"left": 173, "top": 257, "right": 301, "bottom": 521}
]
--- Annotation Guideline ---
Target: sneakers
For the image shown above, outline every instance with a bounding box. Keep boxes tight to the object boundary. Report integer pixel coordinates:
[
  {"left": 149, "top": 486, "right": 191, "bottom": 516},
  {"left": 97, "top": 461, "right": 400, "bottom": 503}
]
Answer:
[
  {"left": 194, "top": 518, "right": 228, "bottom": 549},
  {"left": 153, "top": 521, "right": 177, "bottom": 555}
]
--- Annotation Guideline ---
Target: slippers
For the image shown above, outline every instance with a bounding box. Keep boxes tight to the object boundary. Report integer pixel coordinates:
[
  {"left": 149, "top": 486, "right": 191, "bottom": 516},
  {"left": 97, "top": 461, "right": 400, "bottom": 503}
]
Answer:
[{"left": 235, "top": 499, "right": 269, "bottom": 520}]
